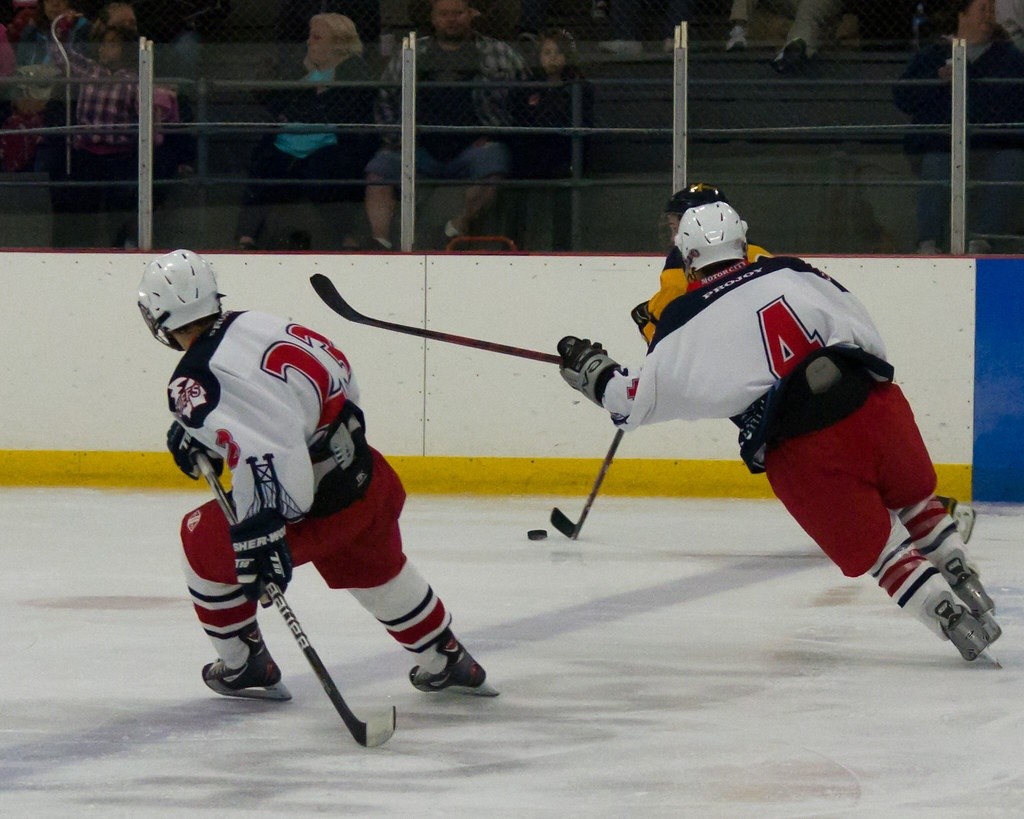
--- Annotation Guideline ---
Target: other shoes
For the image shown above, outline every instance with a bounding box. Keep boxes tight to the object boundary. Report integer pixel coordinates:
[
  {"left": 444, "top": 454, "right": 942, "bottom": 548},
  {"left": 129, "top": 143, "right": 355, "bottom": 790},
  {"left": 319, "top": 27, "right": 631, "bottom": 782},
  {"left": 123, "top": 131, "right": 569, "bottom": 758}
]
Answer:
[
  {"left": 236, "top": 242, "right": 253, "bottom": 250},
  {"left": 352, "top": 237, "right": 386, "bottom": 252}
]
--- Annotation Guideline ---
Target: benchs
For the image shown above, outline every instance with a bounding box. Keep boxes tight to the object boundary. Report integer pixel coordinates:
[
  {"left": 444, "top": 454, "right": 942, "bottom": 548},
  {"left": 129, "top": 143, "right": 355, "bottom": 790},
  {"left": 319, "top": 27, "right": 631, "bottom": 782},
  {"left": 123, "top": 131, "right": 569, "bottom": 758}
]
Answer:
[{"left": 0, "top": 0, "right": 1024, "bottom": 259}]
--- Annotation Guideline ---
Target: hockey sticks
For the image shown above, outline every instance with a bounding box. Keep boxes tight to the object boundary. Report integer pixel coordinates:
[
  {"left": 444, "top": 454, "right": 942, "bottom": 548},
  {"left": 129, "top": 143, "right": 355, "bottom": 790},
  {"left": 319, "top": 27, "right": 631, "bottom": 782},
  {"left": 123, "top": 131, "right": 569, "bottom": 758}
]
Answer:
[
  {"left": 309, "top": 273, "right": 561, "bottom": 364},
  {"left": 193, "top": 450, "right": 398, "bottom": 746},
  {"left": 550, "top": 428, "right": 624, "bottom": 541}
]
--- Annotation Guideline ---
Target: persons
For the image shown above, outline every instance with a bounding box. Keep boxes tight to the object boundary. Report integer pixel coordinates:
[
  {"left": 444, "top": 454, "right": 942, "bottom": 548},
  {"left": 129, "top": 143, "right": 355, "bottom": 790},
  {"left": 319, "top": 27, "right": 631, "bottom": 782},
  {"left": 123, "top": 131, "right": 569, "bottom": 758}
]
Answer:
[
  {"left": 0, "top": 0, "right": 232, "bottom": 249},
  {"left": 243, "top": 0, "right": 593, "bottom": 252},
  {"left": 891, "top": 0, "right": 1024, "bottom": 255},
  {"left": 140, "top": 247, "right": 500, "bottom": 701},
  {"left": 557, "top": 185, "right": 1004, "bottom": 669},
  {"left": 602, "top": 0, "right": 938, "bottom": 74}
]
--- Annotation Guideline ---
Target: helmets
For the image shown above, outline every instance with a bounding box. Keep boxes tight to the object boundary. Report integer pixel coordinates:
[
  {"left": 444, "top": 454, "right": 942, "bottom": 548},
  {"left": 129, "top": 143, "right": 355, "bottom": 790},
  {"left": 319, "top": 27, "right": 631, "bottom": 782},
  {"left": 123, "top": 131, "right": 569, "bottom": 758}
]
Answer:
[
  {"left": 136, "top": 249, "right": 226, "bottom": 351},
  {"left": 674, "top": 200, "right": 747, "bottom": 275},
  {"left": 663, "top": 183, "right": 725, "bottom": 219}
]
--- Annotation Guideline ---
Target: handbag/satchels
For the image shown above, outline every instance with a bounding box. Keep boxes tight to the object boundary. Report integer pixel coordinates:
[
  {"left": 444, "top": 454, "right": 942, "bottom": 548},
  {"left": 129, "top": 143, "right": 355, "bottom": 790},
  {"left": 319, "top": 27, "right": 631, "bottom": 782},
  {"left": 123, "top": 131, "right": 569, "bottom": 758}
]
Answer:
[{"left": 8, "top": 64, "right": 61, "bottom": 114}]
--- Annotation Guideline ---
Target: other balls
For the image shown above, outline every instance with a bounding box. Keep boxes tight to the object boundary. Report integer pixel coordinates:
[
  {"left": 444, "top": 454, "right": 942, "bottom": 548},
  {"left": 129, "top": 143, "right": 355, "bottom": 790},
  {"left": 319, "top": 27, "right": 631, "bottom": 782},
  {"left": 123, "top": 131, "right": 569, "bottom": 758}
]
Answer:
[{"left": 527, "top": 530, "right": 548, "bottom": 540}]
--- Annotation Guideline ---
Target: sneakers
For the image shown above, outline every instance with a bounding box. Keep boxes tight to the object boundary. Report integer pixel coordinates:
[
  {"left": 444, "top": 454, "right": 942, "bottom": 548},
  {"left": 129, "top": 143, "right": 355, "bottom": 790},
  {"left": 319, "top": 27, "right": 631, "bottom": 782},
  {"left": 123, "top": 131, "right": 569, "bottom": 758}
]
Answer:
[
  {"left": 938, "top": 548, "right": 1001, "bottom": 637},
  {"left": 924, "top": 591, "right": 1001, "bottom": 668},
  {"left": 770, "top": 37, "right": 806, "bottom": 73},
  {"left": 935, "top": 495, "right": 976, "bottom": 544},
  {"left": 202, "top": 623, "right": 293, "bottom": 701},
  {"left": 726, "top": 24, "right": 753, "bottom": 51},
  {"left": 409, "top": 628, "right": 499, "bottom": 696}
]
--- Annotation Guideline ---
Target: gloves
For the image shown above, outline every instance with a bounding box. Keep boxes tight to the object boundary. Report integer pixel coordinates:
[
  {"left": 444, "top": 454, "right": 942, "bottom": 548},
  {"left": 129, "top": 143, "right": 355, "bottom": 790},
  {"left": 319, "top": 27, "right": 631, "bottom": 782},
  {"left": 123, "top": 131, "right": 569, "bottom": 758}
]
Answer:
[
  {"left": 166, "top": 420, "right": 222, "bottom": 481},
  {"left": 231, "top": 508, "right": 291, "bottom": 608},
  {"left": 557, "top": 336, "right": 622, "bottom": 407}
]
